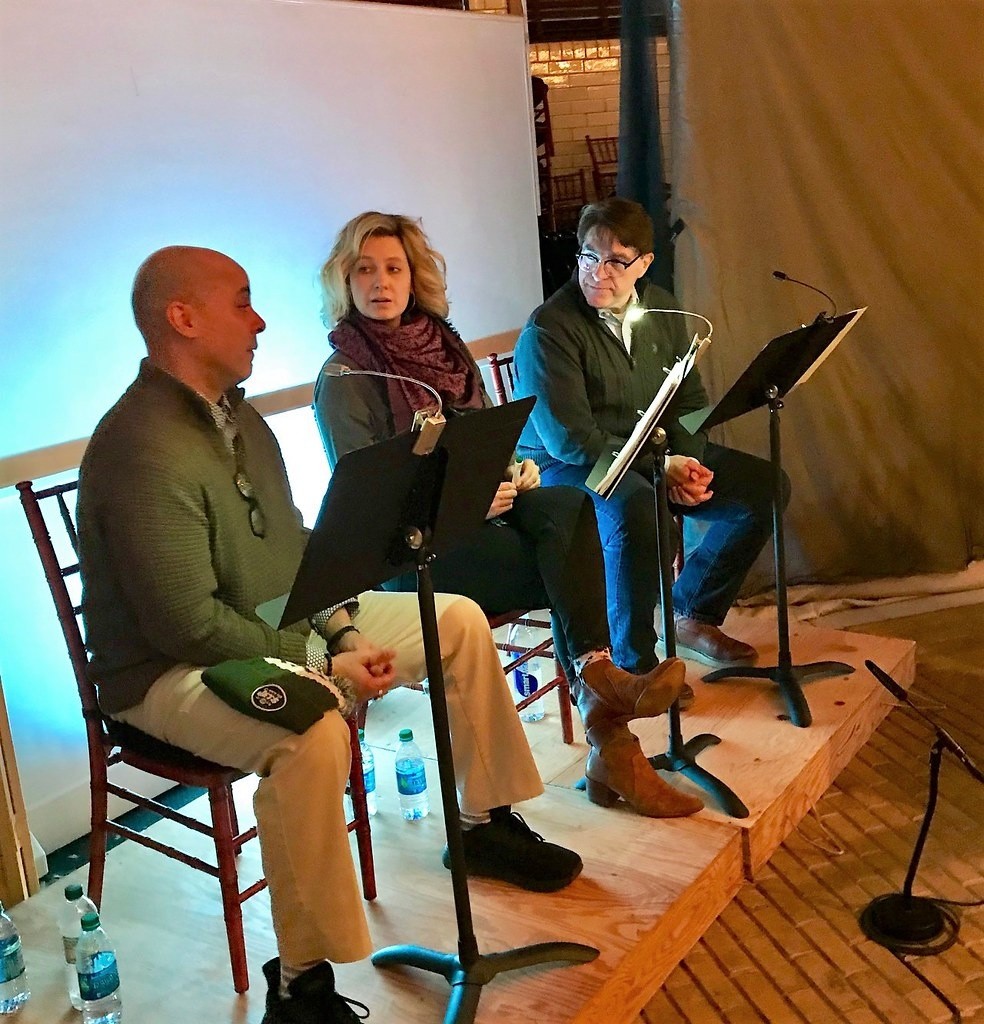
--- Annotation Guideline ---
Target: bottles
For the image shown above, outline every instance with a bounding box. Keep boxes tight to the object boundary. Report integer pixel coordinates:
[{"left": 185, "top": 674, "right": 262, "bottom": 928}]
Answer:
[
  {"left": 509, "top": 612, "right": 544, "bottom": 722},
  {"left": 0, "top": 899, "right": 30, "bottom": 1016},
  {"left": 395, "top": 729, "right": 431, "bottom": 821},
  {"left": 350, "top": 727, "right": 376, "bottom": 818},
  {"left": 58, "top": 885, "right": 109, "bottom": 1011},
  {"left": 73, "top": 913, "right": 122, "bottom": 1024}
]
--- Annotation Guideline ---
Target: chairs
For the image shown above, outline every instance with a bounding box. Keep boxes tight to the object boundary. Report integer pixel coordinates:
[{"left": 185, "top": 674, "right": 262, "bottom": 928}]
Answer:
[
  {"left": 584, "top": 134, "right": 622, "bottom": 199},
  {"left": 547, "top": 167, "right": 588, "bottom": 234},
  {"left": 356, "top": 601, "right": 581, "bottom": 744},
  {"left": 489, "top": 351, "right": 685, "bottom": 581},
  {"left": 15, "top": 476, "right": 377, "bottom": 996}
]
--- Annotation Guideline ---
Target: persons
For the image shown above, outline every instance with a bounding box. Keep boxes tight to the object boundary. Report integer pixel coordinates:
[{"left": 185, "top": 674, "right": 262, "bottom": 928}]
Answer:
[
  {"left": 511, "top": 194, "right": 792, "bottom": 708},
  {"left": 75, "top": 246, "right": 584, "bottom": 1024},
  {"left": 314, "top": 212, "right": 703, "bottom": 821}
]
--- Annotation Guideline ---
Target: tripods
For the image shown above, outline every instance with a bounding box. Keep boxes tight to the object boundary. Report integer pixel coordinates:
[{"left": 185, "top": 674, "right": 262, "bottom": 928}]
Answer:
[{"left": 277, "top": 312, "right": 858, "bottom": 1023}]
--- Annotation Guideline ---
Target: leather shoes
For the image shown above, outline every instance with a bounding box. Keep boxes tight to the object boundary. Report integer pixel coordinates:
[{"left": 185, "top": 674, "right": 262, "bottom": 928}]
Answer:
[
  {"left": 631, "top": 667, "right": 695, "bottom": 707},
  {"left": 658, "top": 613, "right": 759, "bottom": 668}
]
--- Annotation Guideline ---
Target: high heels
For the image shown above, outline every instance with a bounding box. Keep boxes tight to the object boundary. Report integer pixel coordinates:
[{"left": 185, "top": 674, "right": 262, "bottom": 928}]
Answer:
[
  {"left": 570, "top": 657, "right": 686, "bottom": 745},
  {"left": 584, "top": 734, "right": 705, "bottom": 819}
]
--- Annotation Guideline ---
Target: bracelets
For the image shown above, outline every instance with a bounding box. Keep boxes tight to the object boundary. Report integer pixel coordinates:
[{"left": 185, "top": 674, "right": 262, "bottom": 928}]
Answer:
[
  {"left": 325, "top": 653, "right": 333, "bottom": 676},
  {"left": 327, "top": 626, "right": 360, "bottom": 652}
]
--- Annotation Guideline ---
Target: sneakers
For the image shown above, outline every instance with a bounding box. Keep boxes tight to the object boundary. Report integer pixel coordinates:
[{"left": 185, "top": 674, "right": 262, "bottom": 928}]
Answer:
[
  {"left": 441, "top": 804, "right": 583, "bottom": 893},
  {"left": 261, "top": 956, "right": 370, "bottom": 1024}
]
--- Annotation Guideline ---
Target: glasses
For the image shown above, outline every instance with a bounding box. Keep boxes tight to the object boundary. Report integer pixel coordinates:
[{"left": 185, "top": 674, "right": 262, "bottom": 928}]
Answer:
[
  {"left": 575, "top": 248, "right": 642, "bottom": 278},
  {"left": 232, "top": 470, "right": 267, "bottom": 538}
]
